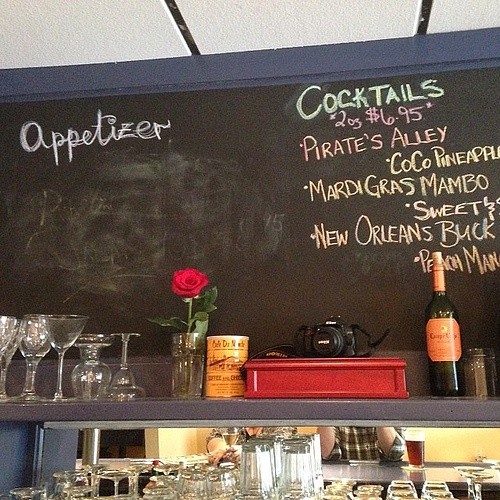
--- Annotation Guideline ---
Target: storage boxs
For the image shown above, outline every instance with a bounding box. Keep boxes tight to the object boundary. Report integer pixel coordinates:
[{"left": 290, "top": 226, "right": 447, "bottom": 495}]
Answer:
[{"left": 245, "top": 355, "right": 411, "bottom": 400}]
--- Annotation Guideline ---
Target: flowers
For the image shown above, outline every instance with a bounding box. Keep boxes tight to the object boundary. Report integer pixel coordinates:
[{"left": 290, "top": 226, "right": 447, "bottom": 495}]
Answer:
[{"left": 139, "top": 265, "right": 218, "bottom": 392}]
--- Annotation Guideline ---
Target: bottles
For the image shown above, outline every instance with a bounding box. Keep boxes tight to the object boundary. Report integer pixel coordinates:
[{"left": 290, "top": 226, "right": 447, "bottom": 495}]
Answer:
[{"left": 425, "top": 252, "right": 466, "bottom": 396}]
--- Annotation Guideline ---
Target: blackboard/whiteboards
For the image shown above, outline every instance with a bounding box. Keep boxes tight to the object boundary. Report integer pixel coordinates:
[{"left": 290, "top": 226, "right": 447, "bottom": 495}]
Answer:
[{"left": 0, "top": 25, "right": 500, "bottom": 429}]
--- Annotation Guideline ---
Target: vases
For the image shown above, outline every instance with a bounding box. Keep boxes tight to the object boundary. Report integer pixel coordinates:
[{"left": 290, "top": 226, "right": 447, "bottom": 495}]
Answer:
[{"left": 169, "top": 331, "right": 206, "bottom": 398}]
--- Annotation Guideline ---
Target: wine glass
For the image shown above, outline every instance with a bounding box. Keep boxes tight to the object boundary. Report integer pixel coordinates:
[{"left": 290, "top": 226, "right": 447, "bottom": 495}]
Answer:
[
  {"left": 19, "top": 314, "right": 143, "bottom": 402},
  {"left": 0, "top": 316, "right": 27, "bottom": 402},
  {"left": 10, "top": 462, "right": 178, "bottom": 500},
  {"left": 220, "top": 427, "right": 245, "bottom": 449},
  {"left": 455, "top": 466, "right": 495, "bottom": 500}
]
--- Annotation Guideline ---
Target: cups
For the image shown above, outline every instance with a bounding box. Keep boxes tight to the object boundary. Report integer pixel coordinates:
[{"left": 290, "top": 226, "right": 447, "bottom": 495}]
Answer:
[
  {"left": 404, "top": 431, "right": 425, "bottom": 467},
  {"left": 325, "top": 480, "right": 459, "bottom": 500},
  {"left": 179, "top": 434, "right": 325, "bottom": 500},
  {"left": 464, "top": 348, "right": 500, "bottom": 397}
]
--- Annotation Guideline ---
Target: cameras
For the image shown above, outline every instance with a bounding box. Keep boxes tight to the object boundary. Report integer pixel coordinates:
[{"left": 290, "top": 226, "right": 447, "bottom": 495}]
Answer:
[{"left": 295, "top": 316, "right": 374, "bottom": 357}]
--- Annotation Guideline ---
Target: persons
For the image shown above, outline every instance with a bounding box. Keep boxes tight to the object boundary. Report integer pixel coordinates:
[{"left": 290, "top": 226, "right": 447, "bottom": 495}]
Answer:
[
  {"left": 317, "top": 427, "right": 406, "bottom": 463},
  {"left": 206, "top": 427, "right": 298, "bottom": 469}
]
